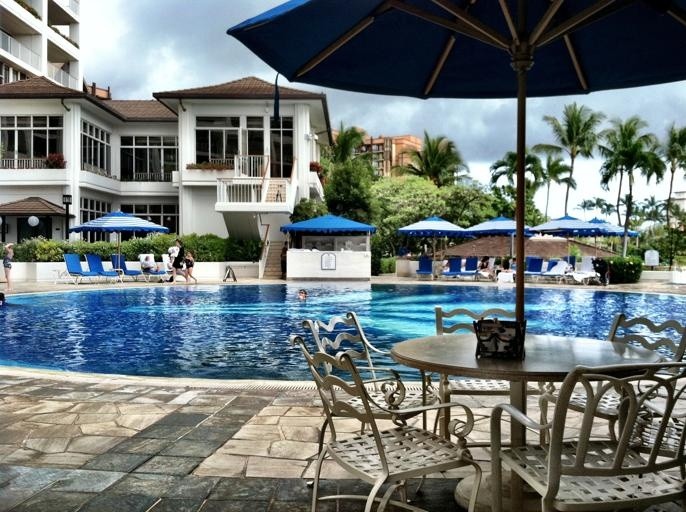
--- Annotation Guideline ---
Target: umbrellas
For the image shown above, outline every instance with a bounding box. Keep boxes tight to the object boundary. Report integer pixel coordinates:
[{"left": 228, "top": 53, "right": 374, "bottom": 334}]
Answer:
[
  {"left": 573, "top": 215, "right": 637, "bottom": 274},
  {"left": 529, "top": 212, "right": 599, "bottom": 280},
  {"left": 464, "top": 211, "right": 532, "bottom": 267},
  {"left": 67, "top": 208, "right": 171, "bottom": 283},
  {"left": 395, "top": 215, "right": 470, "bottom": 270},
  {"left": 224, "top": 0, "right": 686, "bottom": 358}
]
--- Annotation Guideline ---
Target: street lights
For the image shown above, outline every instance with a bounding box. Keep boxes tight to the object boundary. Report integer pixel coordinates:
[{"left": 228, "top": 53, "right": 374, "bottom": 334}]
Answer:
[
  {"left": 62, "top": 194, "right": 72, "bottom": 242},
  {"left": 27, "top": 214, "right": 39, "bottom": 236}
]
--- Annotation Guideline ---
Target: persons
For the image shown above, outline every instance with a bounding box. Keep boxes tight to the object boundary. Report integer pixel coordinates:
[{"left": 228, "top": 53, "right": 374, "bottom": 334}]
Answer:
[
  {"left": 171, "top": 238, "right": 191, "bottom": 284},
  {"left": 398, "top": 244, "right": 410, "bottom": 258},
  {"left": 2, "top": 240, "right": 15, "bottom": 289},
  {"left": 297, "top": 288, "right": 307, "bottom": 301},
  {"left": 477, "top": 255, "right": 493, "bottom": 278},
  {"left": 511, "top": 256, "right": 517, "bottom": 271},
  {"left": 499, "top": 259, "right": 511, "bottom": 282},
  {"left": 184, "top": 251, "right": 197, "bottom": 283},
  {"left": 141, "top": 255, "right": 160, "bottom": 273}
]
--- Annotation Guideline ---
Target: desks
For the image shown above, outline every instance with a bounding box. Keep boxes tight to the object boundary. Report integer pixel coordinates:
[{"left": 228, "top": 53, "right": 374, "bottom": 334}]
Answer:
[{"left": 380, "top": 333, "right": 667, "bottom": 511}]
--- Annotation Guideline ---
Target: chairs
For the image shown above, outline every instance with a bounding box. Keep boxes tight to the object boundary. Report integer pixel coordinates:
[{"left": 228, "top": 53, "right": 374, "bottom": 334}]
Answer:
[
  {"left": 301, "top": 312, "right": 441, "bottom": 491},
  {"left": 61, "top": 250, "right": 192, "bottom": 286},
  {"left": 286, "top": 335, "right": 482, "bottom": 511},
  {"left": 436, "top": 306, "right": 547, "bottom": 453},
  {"left": 415, "top": 255, "right": 598, "bottom": 283},
  {"left": 491, "top": 364, "right": 685, "bottom": 511},
  {"left": 539, "top": 314, "right": 684, "bottom": 487}
]
